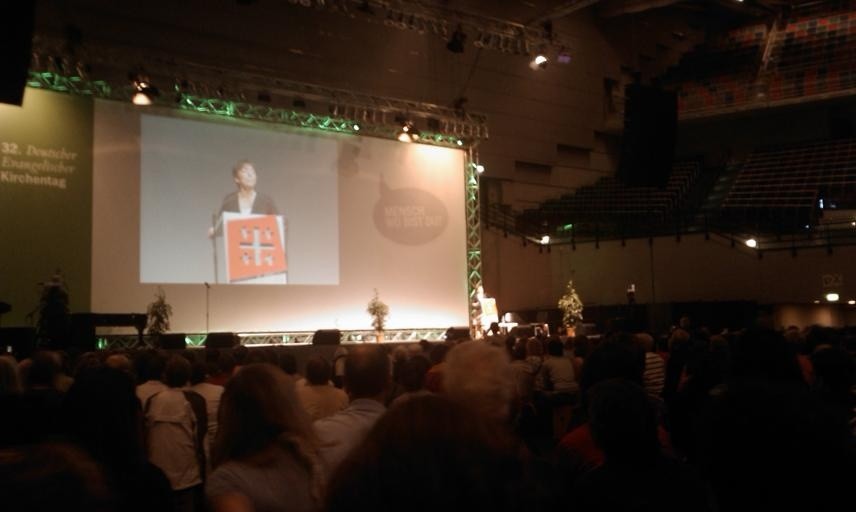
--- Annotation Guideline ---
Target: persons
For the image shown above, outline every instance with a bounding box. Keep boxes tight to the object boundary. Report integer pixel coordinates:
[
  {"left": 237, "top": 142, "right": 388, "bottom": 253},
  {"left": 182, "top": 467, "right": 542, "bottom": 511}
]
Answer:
[
  {"left": 0, "top": 322, "right": 856, "bottom": 512},
  {"left": 208, "top": 160, "right": 276, "bottom": 238},
  {"left": 471, "top": 286, "right": 489, "bottom": 307}
]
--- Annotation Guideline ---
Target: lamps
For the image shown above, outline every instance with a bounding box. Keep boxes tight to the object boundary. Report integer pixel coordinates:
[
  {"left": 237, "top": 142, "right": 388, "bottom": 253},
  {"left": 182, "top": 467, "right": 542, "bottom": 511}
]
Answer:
[
  {"left": 16, "top": 46, "right": 471, "bottom": 150},
  {"left": 301, "top": 1, "right": 575, "bottom": 69}
]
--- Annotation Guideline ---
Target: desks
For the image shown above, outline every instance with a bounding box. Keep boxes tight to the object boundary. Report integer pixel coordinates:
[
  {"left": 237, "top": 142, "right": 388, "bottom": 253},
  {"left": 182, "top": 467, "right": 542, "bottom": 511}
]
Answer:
[{"left": 68, "top": 311, "right": 150, "bottom": 351}]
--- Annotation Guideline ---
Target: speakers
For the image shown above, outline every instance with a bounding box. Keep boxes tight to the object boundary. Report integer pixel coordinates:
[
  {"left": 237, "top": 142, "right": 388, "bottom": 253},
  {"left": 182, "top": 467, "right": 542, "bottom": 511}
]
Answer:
[
  {"left": 312, "top": 330, "right": 342, "bottom": 345},
  {"left": 207, "top": 333, "right": 234, "bottom": 348},
  {"left": 159, "top": 333, "right": 186, "bottom": 349},
  {"left": 0, "top": 0, "right": 36, "bottom": 106},
  {"left": 618, "top": 83, "right": 677, "bottom": 190},
  {"left": 445, "top": 329, "right": 470, "bottom": 342}
]
